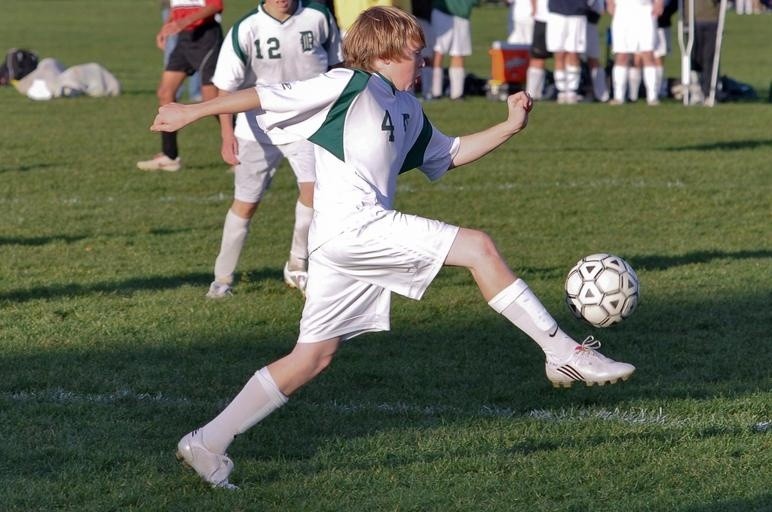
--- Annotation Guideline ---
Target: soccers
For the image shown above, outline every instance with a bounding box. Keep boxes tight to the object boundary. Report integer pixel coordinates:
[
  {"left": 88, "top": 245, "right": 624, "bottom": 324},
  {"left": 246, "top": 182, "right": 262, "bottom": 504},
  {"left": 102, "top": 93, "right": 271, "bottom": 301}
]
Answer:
[{"left": 564, "top": 254, "right": 639, "bottom": 329}]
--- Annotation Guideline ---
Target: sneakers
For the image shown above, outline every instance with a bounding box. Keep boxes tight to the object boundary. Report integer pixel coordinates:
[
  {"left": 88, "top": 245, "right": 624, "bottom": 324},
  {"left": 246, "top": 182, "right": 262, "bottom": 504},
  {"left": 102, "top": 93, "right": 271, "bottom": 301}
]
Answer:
[
  {"left": 542, "top": 341, "right": 636, "bottom": 389},
  {"left": 137, "top": 152, "right": 181, "bottom": 172},
  {"left": 172, "top": 428, "right": 240, "bottom": 494},
  {"left": 421, "top": 89, "right": 716, "bottom": 106},
  {"left": 206, "top": 282, "right": 230, "bottom": 299},
  {"left": 283, "top": 261, "right": 309, "bottom": 298}
]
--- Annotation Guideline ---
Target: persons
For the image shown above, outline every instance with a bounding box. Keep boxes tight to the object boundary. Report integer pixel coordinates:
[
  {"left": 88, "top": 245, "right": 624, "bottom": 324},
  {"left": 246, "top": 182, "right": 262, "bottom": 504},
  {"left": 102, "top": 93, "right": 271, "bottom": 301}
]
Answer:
[
  {"left": 206, "top": 0, "right": 346, "bottom": 299},
  {"left": 177, "top": 7, "right": 634, "bottom": 494},
  {"left": 136, "top": 0, "right": 238, "bottom": 172},
  {"left": 161, "top": 1, "right": 203, "bottom": 104}
]
